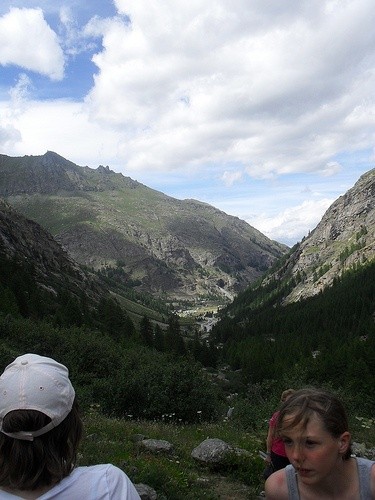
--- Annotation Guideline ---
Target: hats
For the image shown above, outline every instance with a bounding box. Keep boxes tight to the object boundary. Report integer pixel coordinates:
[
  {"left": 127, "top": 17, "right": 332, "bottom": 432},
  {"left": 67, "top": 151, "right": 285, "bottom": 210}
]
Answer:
[{"left": 0, "top": 354, "right": 76, "bottom": 441}]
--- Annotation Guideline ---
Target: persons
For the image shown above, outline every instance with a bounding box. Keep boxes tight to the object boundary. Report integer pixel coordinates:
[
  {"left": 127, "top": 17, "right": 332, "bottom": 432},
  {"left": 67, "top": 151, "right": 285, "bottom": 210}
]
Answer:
[
  {"left": 0, "top": 352, "right": 142, "bottom": 500},
  {"left": 264, "top": 388, "right": 375, "bottom": 500},
  {"left": 264, "top": 389, "right": 296, "bottom": 478}
]
